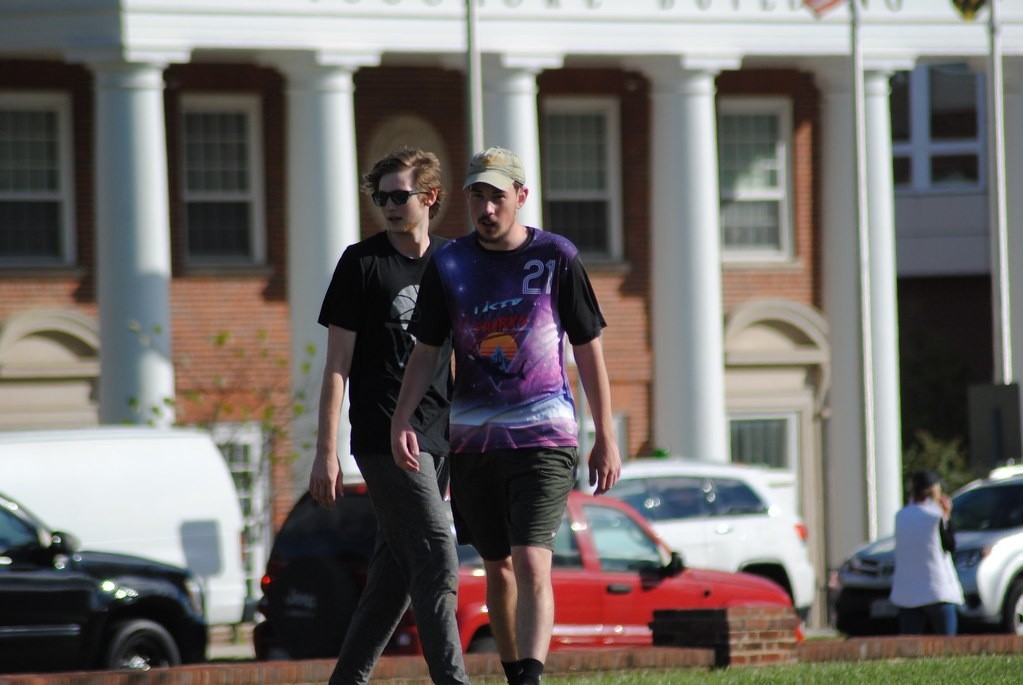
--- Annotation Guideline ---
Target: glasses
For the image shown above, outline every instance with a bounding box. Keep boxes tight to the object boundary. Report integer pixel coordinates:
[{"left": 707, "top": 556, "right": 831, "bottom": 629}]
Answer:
[{"left": 371, "top": 189, "right": 428, "bottom": 208}]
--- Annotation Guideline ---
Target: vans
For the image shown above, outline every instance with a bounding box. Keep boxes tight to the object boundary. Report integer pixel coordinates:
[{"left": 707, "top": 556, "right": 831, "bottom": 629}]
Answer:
[{"left": 0, "top": 430, "right": 246, "bottom": 630}]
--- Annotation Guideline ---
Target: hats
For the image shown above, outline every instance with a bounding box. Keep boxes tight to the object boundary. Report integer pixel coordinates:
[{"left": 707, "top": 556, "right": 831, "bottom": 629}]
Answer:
[
  {"left": 911, "top": 470, "right": 946, "bottom": 492},
  {"left": 463, "top": 146, "right": 525, "bottom": 191}
]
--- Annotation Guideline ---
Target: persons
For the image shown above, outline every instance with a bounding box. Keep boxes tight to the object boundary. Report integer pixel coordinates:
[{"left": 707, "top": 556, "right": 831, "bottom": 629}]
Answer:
[
  {"left": 889, "top": 470, "right": 965, "bottom": 636},
  {"left": 309, "top": 148, "right": 470, "bottom": 685},
  {"left": 391, "top": 145, "right": 622, "bottom": 685}
]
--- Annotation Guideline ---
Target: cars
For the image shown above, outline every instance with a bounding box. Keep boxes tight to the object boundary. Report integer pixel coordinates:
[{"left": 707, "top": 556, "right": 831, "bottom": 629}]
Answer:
[{"left": 0, "top": 497, "right": 213, "bottom": 673}]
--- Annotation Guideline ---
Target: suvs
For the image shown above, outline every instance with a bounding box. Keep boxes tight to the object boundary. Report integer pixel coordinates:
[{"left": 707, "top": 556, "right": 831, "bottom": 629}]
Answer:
[
  {"left": 833, "top": 461, "right": 1023, "bottom": 637},
  {"left": 252, "top": 480, "right": 800, "bottom": 663},
  {"left": 445, "top": 459, "right": 816, "bottom": 629}
]
